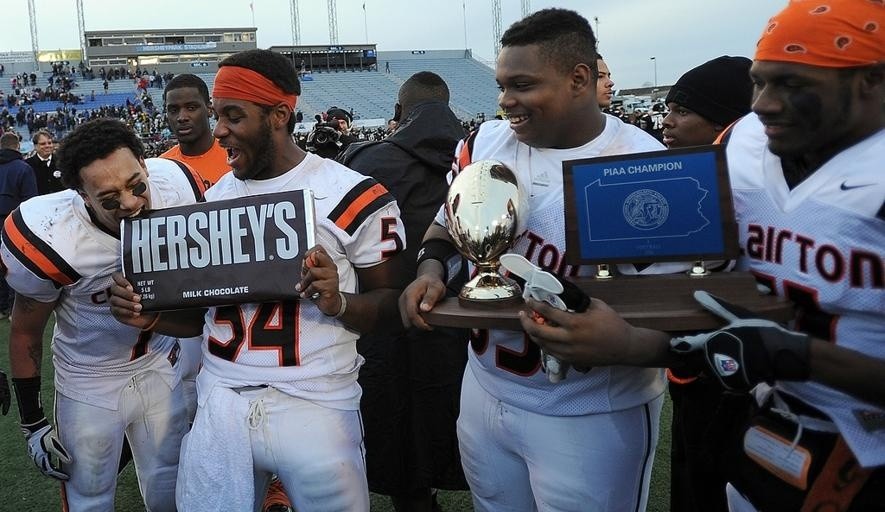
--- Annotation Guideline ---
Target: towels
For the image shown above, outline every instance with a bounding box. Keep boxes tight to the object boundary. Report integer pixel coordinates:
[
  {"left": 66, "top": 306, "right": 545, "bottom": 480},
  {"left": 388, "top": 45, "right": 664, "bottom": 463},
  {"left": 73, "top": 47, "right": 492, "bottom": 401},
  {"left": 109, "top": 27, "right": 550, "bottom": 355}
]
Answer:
[{"left": 175, "top": 382, "right": 256, "bottom": 512}]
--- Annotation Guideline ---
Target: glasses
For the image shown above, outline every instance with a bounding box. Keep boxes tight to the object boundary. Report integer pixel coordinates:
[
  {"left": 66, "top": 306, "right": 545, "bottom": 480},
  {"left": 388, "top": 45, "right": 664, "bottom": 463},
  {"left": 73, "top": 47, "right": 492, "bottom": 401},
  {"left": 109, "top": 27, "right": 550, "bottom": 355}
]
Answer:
[{"left": 101, "top": 182, "right": 147, "bottom": 211}]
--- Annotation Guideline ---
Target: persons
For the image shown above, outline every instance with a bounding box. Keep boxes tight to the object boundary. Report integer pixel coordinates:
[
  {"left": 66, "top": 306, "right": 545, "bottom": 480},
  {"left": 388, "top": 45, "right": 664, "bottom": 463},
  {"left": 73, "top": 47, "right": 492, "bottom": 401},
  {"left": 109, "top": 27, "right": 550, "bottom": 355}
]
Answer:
[{"left": 0, "top": 0, "right": 884, "bottom": 511}]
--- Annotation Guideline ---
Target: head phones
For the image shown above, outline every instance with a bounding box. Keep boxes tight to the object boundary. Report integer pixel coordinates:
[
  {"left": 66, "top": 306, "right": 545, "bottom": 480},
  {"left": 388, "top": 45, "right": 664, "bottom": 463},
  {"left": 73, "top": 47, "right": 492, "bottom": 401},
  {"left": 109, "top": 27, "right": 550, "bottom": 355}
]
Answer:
[{"left": 331, "top": 117, "right": 353, "bottom": 128}]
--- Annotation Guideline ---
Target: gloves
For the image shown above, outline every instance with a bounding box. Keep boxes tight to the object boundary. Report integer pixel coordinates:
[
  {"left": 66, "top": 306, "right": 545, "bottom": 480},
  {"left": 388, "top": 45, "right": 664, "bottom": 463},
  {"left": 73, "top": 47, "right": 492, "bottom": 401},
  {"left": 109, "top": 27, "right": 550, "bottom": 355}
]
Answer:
[
  {"left": 667, "top": 290, "right": 812, "bottom": 402},
  {"left": 18, "top": 418, "right": 74, "bottom": 483}
]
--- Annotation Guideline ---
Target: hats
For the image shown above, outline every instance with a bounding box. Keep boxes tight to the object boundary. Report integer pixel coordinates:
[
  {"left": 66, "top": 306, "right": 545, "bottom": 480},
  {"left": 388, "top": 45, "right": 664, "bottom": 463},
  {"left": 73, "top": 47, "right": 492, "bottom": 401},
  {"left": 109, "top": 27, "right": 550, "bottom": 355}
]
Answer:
[{"left": 665, "top": 55, "right": 753, "bottom": 127}]
